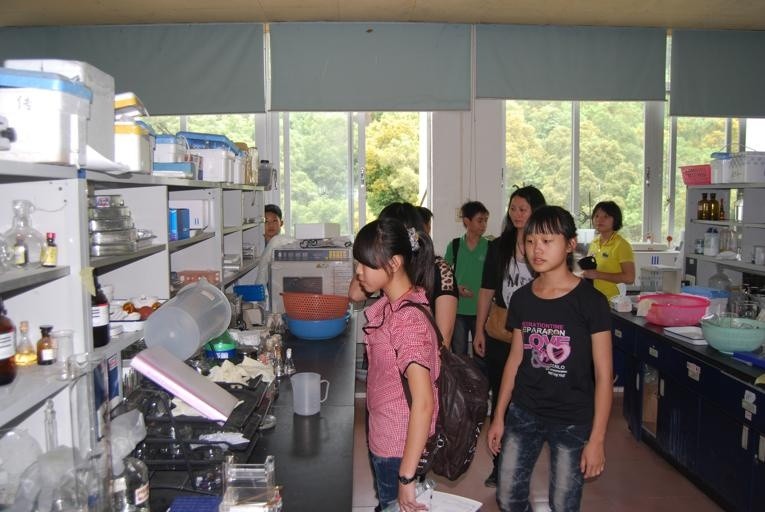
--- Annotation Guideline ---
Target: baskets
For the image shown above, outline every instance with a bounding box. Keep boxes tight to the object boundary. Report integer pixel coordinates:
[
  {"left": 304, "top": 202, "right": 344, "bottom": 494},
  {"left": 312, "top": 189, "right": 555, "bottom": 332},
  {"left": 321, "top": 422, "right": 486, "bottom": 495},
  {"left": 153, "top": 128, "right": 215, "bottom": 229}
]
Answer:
[{"left": 279, "top": 292, "right": 349, "bottom": 320}]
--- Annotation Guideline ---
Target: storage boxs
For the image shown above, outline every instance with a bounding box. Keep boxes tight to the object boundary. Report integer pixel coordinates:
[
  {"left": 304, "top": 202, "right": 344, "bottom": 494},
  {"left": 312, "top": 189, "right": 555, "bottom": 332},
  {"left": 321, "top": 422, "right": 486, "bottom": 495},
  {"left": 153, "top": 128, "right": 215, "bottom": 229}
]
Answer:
[
  {"left": 710, "top": 152, "right": 765, "bottom": 183},
  {"left": 0, "top": 58, "right": 247, "bottom": 185}
]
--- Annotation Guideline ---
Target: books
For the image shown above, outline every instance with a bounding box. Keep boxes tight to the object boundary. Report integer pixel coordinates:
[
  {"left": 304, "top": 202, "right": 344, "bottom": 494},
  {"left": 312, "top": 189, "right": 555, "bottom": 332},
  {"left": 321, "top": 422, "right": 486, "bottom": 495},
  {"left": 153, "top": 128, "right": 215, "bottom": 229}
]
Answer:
[{"left": 129, "top": 344, "right": 239, "bottom": 427}]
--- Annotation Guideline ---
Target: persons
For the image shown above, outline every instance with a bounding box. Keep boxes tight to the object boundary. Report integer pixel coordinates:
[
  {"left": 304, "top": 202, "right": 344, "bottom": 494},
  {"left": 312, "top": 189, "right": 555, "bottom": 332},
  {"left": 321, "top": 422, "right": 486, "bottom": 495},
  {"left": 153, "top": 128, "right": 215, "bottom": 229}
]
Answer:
[
  {"left": 264, "top": 205, "right": 283, "bottom": 248},
  {"left": 472, "top": 186, "right": 547, "bottom": 488},
  {"left": 445, "top": 201, "right": 494, "bottom": 416},
  {"left": 487, "top": 204, "right": 614, "bottom": 512},
  {"left": 582, "top": 200, "right": 636, "bottom": 387},
  {"left": 349, "top": 201, "right": 459, "bottom": 512}
]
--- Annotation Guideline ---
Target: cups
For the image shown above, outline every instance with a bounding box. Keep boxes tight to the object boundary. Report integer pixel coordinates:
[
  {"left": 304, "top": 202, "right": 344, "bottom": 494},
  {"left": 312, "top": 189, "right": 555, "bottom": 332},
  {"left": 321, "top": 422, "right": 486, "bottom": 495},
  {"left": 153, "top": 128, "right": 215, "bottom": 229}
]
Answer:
[{"left": 51, "top": 328, "right": 74, "bottom": 364}]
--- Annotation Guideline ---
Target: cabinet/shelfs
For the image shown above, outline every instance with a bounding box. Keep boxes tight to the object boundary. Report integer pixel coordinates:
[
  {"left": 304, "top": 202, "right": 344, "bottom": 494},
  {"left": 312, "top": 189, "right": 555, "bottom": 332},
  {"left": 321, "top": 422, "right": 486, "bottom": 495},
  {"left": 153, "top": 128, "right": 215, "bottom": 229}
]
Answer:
[
  {"left": 0, "top": 159, "right": 266, "bottom": 458},
  {"left": 682, "top": 182, "right": 762, "bottom": 294},
  {"left": 611, "top": 310, "right": 764, "bottom": 511}
]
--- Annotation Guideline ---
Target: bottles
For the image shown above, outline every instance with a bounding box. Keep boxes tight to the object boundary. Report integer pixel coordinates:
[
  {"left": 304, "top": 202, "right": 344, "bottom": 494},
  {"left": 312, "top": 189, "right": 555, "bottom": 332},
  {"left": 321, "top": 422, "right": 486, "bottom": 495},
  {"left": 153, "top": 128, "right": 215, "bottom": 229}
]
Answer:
[
  {"left": 205, "top": 325, "right": 238, "bottom": 367},
  {"left": 708, "top": 264, "right": 730, "bottom": 293},
  {"left": 41, "top": 232, "right": 58, "bottom": 267},
  {"left": 703, "top": 226, "right": 721, "bottom": 257},
  {"left": 15, "top": 237, "right": 28, "bottom": 266},
  {"left": 0, "top": 299, "right": 56, "bottom": 386},
  {"left": 696, "top": 193, "right": 724, "bottom": 220},
  {"left": 274, "top": 349, "right": 295, "bottom": 377},
  {"left": 378, "top": 479, "right": 436, "bottom": 512},
  {"left": 696, "top": 239, "right": 704, "bottom": 254},
  {"left": 728, "top": 285, "right": 744, "bottom": 314},
  {"left": 90, "top": 276, "right": 111, "bottom": 349},
  {"left": 258, "top": 159, "right": 270, "bottom": 186}
]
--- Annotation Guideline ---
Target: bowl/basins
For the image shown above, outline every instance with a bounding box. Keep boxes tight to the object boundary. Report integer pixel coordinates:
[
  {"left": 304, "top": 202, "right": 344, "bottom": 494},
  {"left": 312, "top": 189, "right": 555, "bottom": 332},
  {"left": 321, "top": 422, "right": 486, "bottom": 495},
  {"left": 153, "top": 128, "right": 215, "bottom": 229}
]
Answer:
[
  {"left": 701, "top": 316, "right": 765, "bottom": 355},
  {"left": 636, "top": 293, "right": 712, "bottom": 327}
]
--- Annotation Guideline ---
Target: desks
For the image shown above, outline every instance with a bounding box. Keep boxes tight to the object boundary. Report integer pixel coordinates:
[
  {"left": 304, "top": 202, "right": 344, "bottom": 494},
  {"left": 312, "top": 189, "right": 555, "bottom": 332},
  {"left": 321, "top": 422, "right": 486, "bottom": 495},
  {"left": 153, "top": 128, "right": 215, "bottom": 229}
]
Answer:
[{"left": 149, "top": 314, "right": 357, "bottom": 512}]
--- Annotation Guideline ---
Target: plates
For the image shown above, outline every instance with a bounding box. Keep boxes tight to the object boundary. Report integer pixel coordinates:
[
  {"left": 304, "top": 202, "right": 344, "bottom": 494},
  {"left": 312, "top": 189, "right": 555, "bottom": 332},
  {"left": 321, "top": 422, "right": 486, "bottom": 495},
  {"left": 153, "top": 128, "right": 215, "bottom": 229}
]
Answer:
[{"left": 282, "top": 312, "right": 351, "bottom": 340}]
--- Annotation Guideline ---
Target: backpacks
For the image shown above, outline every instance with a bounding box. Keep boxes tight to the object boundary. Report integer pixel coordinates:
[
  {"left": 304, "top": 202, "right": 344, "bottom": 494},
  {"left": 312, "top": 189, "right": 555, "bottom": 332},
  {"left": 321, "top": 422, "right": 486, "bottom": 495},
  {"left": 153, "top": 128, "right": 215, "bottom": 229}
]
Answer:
[{"left": 392, "top": 302, "right": 489, "bottom": 481}]
